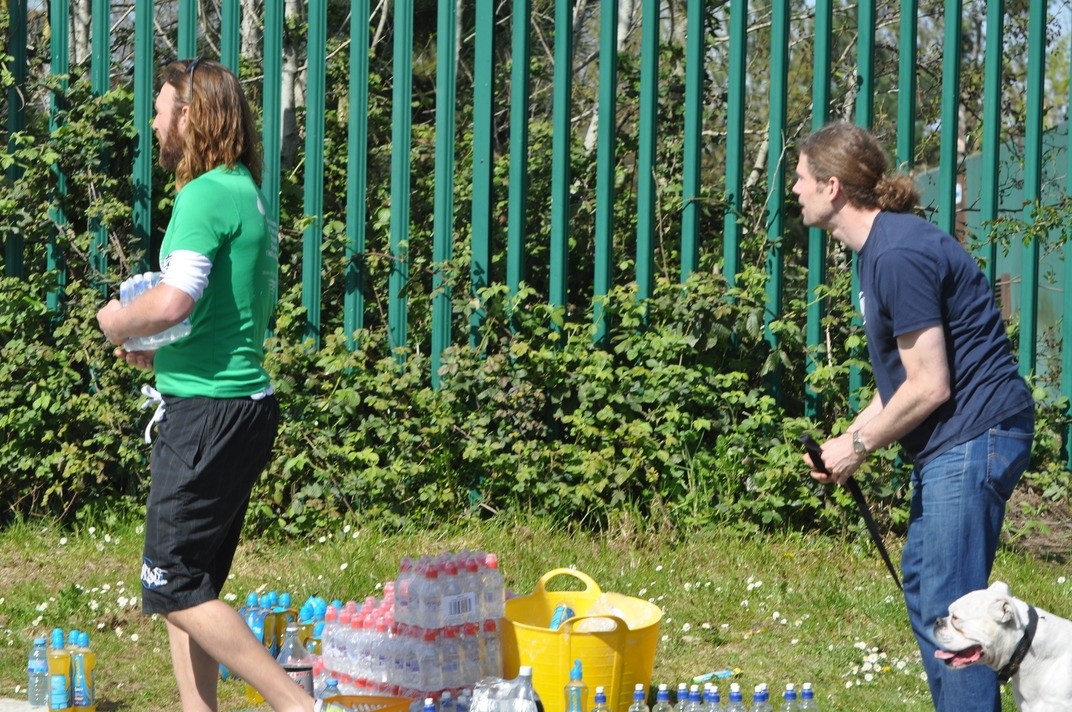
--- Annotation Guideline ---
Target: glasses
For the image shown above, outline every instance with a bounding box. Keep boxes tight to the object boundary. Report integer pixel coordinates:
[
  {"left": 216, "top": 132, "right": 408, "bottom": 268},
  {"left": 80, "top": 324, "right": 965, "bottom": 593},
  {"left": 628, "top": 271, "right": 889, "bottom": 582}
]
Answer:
[{"left": 184, "top": 53, "right": 208, "bottom": 103}]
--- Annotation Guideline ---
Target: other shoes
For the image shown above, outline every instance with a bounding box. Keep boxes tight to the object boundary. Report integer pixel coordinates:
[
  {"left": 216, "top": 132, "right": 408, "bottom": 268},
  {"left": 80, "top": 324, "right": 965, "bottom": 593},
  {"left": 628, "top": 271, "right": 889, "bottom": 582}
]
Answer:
[{"left": 313, "top": 698, "right": 345, "bottom": 712}]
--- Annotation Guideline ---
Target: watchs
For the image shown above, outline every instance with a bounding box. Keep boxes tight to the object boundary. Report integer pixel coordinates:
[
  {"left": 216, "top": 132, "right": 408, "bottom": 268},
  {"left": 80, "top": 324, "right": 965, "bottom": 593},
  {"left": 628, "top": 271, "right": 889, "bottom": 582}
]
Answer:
[{"left": 852, "top": 430, "right": 872, "bottom": 457}]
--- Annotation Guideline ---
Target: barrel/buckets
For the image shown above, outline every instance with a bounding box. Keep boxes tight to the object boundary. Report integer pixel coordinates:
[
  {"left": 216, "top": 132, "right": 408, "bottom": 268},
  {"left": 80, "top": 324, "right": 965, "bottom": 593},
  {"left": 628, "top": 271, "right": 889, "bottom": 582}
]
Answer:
[{"left": 501, "top": 567, "right": 662, "bottom": 712}]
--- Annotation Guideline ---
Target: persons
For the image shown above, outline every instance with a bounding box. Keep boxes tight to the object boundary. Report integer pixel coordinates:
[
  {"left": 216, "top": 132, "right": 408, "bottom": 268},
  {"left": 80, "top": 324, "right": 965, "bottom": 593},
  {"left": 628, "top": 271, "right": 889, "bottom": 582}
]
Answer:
[
  {"left": 97, "top": 57, "right": 317, "bottom": 712},
  {"left": 791, "top": 122, "right": 1036, "bottom": 712}
]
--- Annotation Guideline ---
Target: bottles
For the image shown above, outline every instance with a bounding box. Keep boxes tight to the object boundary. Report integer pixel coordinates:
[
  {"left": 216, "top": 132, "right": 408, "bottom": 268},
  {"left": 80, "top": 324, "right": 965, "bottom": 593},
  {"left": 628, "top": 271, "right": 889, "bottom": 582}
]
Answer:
[
  {"left": 27, "top": 637, "right": 49, "bottom": 704},
  {"left": 241, "top": 550, "right": 544, "bottom": 712},
  {"left": 629, "top": 684, "right": 650, "bottom": 712},
  {"left": 119, "top": 272, "right": 192, "bottom": 355},
  {"left": 725, "top": 683, "right": 748, "bottom": 712},
  {"left": 779, "top": 684, "right": 799, "bottom": 712},
  {"left": 46, "top": 627, "right": 95, "bottom": 712},
  {"left": 750, "top": 682, "right": 775, "bottom": 712},
  {"left": 801, "top": 682, "right": 818, "bottom": 712},
  {"left": 549, "top": 601, "right": 576, "bottom": 629},
  {"left": 591, "top": 686, "right": 609, "bottom": 712},
  {"left": 674, "top": 683, "right": 724, "bottom": 712},
  {"left": 563, "top": 658, "right": 584, "bottom": 712},
  {"left": 652, "top": 683, "right": 673, "bottom": 712}
]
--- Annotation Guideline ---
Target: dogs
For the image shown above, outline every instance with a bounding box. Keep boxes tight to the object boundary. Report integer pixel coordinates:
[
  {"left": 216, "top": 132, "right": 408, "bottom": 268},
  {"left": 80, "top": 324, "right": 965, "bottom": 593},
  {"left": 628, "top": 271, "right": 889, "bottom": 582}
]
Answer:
[{"left": 929, "top": 576, "right": 1072, "bottom": 712}]
